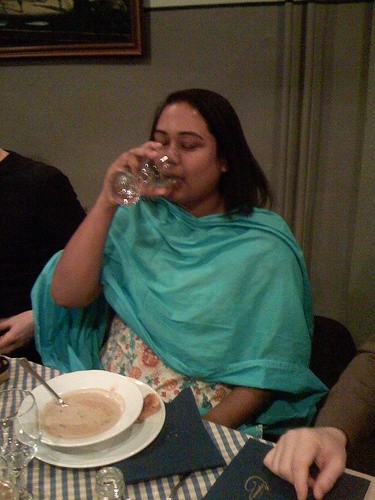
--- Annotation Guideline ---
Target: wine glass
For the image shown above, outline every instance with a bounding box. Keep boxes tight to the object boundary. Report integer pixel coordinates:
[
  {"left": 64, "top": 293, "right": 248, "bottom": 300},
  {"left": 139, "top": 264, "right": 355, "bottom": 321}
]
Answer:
[
  {"left": 0, "top": 388, "right": 40, "bottom": 500},
  {"left": 111, "top": 145, "right": 178, "bottom": 204}
]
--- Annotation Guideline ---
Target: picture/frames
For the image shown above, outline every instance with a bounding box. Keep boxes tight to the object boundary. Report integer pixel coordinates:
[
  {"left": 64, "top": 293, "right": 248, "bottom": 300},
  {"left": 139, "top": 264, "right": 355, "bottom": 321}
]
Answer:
[{"left": 0, "top": 0, "right": 144, "bottom": 60}]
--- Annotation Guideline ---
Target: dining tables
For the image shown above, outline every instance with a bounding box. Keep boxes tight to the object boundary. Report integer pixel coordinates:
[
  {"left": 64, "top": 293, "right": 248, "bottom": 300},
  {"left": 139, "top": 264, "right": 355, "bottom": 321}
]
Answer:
[{"left": 0, "top": 352, "right": 375, "bottom": 500}]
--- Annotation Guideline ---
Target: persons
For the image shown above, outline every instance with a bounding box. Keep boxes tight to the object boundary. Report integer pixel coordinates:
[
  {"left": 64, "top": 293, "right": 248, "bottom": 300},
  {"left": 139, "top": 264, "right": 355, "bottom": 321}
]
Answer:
[
  {"left": 0, "top": 147, "right": 91, "bottom": 368},
  {"left": 263, "top": 332, "right": 374, "bottom": 500},
  {"left": 30, "top": 89, "right": 329, "bottom": 447}
]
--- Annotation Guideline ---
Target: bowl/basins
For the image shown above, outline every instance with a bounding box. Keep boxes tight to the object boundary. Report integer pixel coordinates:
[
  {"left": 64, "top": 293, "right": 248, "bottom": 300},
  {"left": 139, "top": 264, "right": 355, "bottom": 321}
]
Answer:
[{"left": 17, "top": 370, "right": 144, "bottom": 447}]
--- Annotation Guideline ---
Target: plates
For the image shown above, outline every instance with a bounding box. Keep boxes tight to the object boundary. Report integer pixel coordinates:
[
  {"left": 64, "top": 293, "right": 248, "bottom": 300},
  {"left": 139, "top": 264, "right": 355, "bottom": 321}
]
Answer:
[{"left": 34, "top": 376, "right": 166, "bottom": 468}]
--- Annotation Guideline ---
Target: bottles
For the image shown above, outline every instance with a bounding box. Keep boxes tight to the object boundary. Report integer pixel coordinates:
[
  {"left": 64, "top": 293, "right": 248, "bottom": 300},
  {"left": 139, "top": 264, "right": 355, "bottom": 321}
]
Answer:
[{"left": 95, "top": 467, "right": 125, "bottom": 500}]
